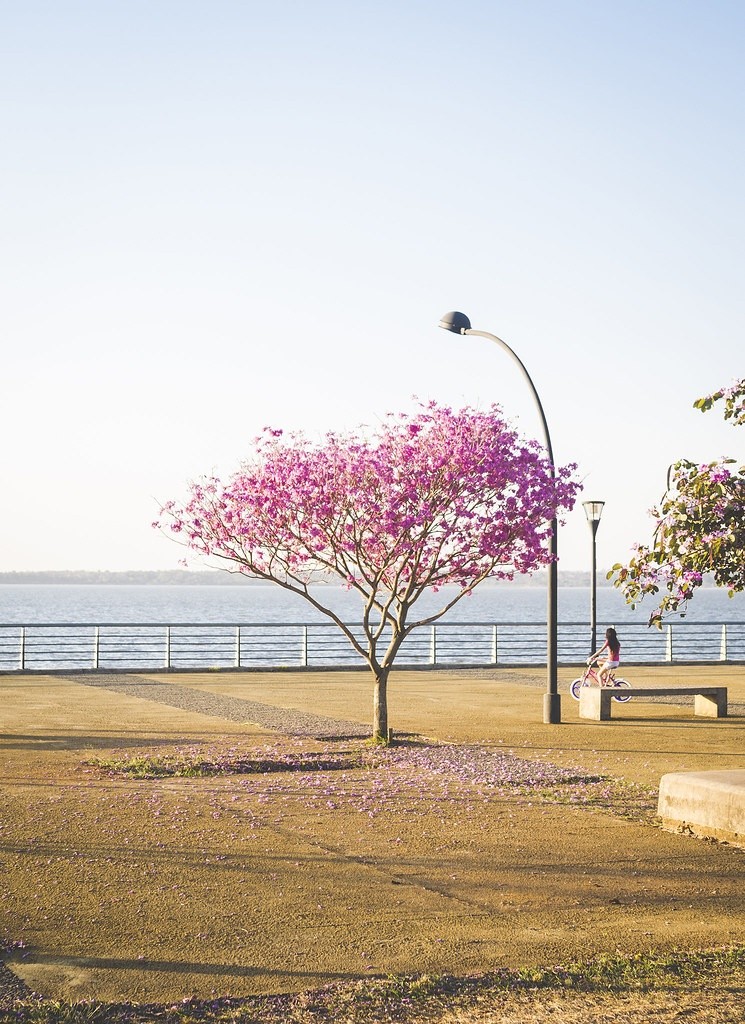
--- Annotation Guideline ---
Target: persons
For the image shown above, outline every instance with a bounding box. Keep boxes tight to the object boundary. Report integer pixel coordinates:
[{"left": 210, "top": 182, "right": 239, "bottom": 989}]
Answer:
[{"left": 587, "top": 628, "right": 620, "bottom": 687}]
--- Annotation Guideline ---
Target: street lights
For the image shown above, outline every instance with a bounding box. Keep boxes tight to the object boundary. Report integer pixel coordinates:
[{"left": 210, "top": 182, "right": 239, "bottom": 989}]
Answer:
[
  {"left": 438, "top": 311, "right": 562, "bottom": 724},
  {"left": 583, "top": 497, "right": 606, "bottom": 667}
]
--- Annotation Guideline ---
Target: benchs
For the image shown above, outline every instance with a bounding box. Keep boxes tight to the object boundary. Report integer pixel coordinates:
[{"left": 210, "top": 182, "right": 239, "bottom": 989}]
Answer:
[{"left": 579, "top": 686, "right": 727, "bottom": 720}]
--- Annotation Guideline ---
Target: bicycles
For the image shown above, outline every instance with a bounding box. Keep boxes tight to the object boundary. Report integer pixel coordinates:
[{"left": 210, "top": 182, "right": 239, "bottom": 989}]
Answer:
[{"left": 570, "top": 656, "right": 633, "bottom": 702}]
